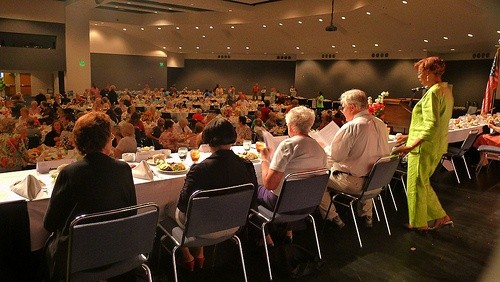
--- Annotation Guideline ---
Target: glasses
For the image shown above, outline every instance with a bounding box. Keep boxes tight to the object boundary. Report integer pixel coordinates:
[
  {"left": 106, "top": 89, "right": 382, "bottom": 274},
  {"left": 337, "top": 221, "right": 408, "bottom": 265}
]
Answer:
[{"left": 111, "top": 135, "right": 115, "bottom": 141}]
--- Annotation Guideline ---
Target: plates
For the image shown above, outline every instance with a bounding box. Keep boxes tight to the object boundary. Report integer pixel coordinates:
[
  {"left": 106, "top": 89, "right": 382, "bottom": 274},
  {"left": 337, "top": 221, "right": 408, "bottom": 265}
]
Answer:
[
  {"left": 157, "top": 164, "right": 190, "bottom": 173},
  {"left": 147, "top": 158, "right": 156, "bottom": 166}
]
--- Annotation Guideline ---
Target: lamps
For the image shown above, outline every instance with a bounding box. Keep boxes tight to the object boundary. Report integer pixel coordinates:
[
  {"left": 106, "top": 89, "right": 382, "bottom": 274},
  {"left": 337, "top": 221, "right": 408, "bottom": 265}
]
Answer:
[{"left": 326, "top": 0, "right": 338, "bottom": 31}]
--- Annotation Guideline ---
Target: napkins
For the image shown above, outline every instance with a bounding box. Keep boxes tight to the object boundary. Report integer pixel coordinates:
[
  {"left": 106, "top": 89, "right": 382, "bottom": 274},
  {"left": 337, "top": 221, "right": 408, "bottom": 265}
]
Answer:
[
  {"left": 132, "top": 160, "right": 155, "bottom": 182},
  {"left": 11, "top": 174, "right": 43, "bottom": 202}
]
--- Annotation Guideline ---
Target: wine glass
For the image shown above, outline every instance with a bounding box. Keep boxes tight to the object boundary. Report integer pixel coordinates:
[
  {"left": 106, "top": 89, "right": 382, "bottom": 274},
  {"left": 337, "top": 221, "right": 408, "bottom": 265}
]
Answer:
[
  {"left": 243, "top": 140, "right": 251, "bottom": 154},
  {"left": 190, "top": 149, "right": 200, "bottom": 164},
  {"left": 256, "top": 141, "right": 265, "bottom": 156},
  {"left": 178, "top": 147, "right": 188, "bottom": 163}
]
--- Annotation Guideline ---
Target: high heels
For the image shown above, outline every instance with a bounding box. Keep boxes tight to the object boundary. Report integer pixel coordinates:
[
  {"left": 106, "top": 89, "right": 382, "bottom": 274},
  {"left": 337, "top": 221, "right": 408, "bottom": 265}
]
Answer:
[
  {"left": 428, "top": 217, "right": 454, "bottom": 232},
  {"left": 403, "top": 224, "right": 428, "bottom": 234},
  {"left": 176, "top": 255, "right": 203, "bottom": 271}
]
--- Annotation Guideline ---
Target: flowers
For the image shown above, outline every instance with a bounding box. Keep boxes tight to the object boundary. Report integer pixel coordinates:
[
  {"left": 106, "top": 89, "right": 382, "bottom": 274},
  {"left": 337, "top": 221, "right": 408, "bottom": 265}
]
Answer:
[{"left": 366, "top": 90, "right": 390, "bottom": 118}]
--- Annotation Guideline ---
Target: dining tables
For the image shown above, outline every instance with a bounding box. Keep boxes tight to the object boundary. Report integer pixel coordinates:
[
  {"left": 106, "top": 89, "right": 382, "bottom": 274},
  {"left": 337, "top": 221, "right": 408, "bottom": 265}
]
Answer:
[
  {"left": 447, "top": 118, "right": 500, "bottom": 143},
  {"left": 0, "top": 143, "right": 268, "bottom": 203}
]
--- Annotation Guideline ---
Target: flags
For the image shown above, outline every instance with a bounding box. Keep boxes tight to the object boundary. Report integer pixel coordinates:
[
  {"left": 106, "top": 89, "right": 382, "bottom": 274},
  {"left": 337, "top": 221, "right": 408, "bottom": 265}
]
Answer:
[{"left": 480, "top": 49, "right": 499, "bottom": 114}]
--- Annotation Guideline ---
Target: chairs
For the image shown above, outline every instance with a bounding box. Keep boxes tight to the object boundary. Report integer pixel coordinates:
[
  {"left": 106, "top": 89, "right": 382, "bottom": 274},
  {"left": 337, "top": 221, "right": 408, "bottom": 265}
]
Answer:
[
  {"left": 247, "top": 169, "right": 331, "bottom": 281},
  {"left": 320, "top": 155, "right": 400, "bottom": 248},
  {"left": 476, "top": 145, "right": 500, "bottom": 176},
  {"left": 438, "top": 129, "right": 479, "bottom": 184},
  {"left": 155, "top": 182, "right": 254, "bottom": 282},
  {"left": 66, "top": 203, "right": 160, "bottom": 282}
]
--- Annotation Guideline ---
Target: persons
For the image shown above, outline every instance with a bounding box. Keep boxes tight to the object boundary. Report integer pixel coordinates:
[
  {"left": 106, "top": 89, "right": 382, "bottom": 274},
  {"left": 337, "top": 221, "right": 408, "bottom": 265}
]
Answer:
[
  {"left": 42, "top": 112, "right": 137, "bottom": 282},
  {"left": 255, "top": 105, "right": 326, "bottom": 248},
  {"left": 0, "top": 81, "right": 350, "bottom": 136},
  {"left": 473, "top": 119, "right": 500, "bottom": 166},
  {"left": 389, "top": 57, "right": 454, "bottom": 233},
  {"left": 174, "top": 118, "right": 259, "bottom": 270},
  {"left": 320, "top": 89, "right": 388, "bottom": 230}
]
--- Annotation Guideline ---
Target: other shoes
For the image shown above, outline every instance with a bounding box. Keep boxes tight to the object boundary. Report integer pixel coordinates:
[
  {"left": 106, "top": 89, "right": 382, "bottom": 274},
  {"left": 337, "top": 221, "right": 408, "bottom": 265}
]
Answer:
[
  {"left": 357, "top": 215, "right": 373, "bottom": 228},
  {"left": 332, "top": 215, "right": 345, "bottom": 231},
  {"left": 283, "top": 235, "right": 293, "bottom": 244},
  {"left": 254, "top": 241, "right": 274, "bottom": 249}
]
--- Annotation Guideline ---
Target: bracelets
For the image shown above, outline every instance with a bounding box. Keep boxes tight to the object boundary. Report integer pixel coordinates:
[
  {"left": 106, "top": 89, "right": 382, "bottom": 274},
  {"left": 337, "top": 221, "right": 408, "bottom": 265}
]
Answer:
[{"left": 261, "top": 159, "right": 270, "bottom": 163}]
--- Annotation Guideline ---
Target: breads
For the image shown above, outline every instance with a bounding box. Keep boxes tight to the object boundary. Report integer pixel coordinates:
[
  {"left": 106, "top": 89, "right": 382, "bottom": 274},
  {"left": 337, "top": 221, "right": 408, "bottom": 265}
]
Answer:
[
  {"left": 57, "top": 164, "right": 70, "bottom": 171},
  {"left": 247, "top": 153, "right": 258, "bottom": 159},
  {"left": 160, "top": 163, "right": 185, "bottom": 171}
]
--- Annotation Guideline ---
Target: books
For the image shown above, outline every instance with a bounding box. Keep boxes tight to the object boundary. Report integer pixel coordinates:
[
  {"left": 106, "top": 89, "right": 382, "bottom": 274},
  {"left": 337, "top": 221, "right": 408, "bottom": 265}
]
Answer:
[
  {"left": 308, "top": 121, "right": 341, "bottom": 157},
  {"left": 262, "top": 129, "right": 290, "bottom": 163}
]
